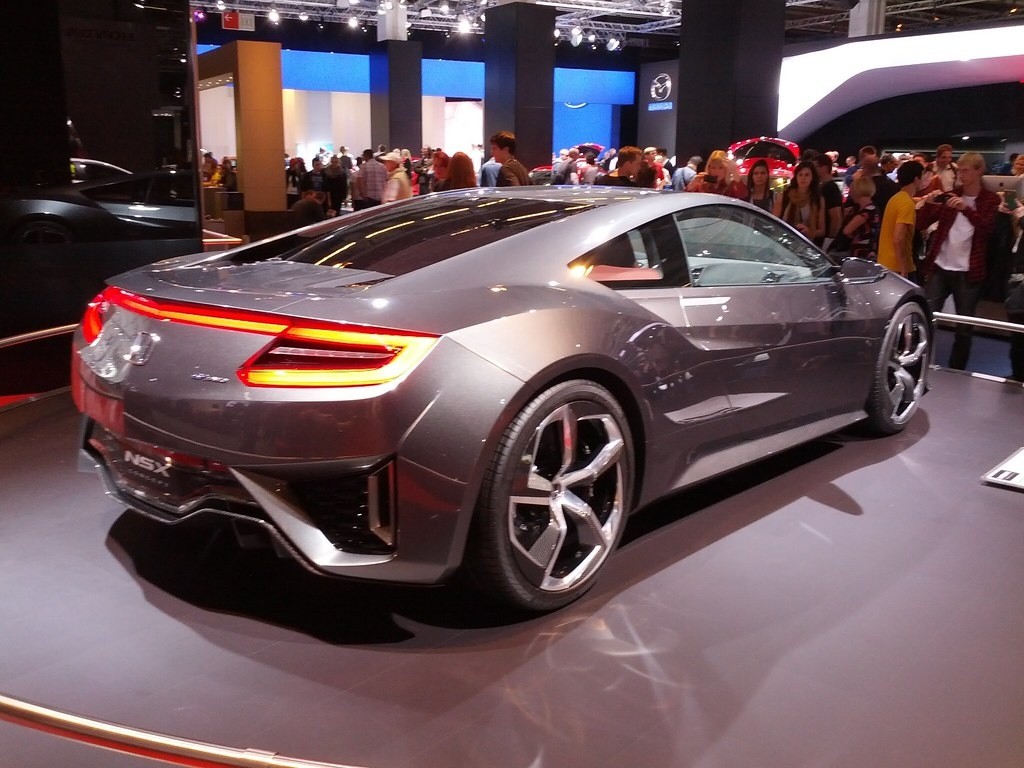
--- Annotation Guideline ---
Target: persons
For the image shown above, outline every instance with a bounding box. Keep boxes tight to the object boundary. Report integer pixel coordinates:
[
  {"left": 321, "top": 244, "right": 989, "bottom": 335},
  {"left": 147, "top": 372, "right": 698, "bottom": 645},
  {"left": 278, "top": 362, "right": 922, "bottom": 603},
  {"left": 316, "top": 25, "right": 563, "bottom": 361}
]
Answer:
[
  {"left": 202, "top": 152, "right": 238, "bottom": 192},
  {"left": 594, "top": 145, "right": 675, "bottom": 190},
  {"left": 479, "top": 131, "right": 530, "bottom": 186},
  {"left": 349, "top": 144, "right": 413, "bottom": 212},
  {"left": 772, "top": 145, "right": 898, "bottom": 264},
  {"left": 985, "top": 152, "right": 1024, "bottom": 303},
  {"left": 414, "top": 145, "right": 479, "bottom": 195},
  {"left": 551, "top": 147, "right": 598, "bottom": 186},
  {"left": 285, "top": 146, "right": 354, "bottom": 221},
  {"left": 878, "top": 144, "right": 1001, "bottom": 371},
  {"left": 671, "top": 150, "right": 779, "bottom": 215}
]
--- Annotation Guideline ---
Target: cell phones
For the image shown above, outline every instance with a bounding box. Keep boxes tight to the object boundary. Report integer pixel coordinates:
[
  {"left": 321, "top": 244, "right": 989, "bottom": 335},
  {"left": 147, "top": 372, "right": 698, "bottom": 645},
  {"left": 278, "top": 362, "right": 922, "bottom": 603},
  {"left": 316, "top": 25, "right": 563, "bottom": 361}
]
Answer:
[
  {"left": 934, "top": 194, "right": 953, "bottom": 203},
  {"left": 703, "top": 175, "right": 718, "bottom": 184},
  {"left": 1003, "top": 189, "right": 1018, "bottom": 211}
]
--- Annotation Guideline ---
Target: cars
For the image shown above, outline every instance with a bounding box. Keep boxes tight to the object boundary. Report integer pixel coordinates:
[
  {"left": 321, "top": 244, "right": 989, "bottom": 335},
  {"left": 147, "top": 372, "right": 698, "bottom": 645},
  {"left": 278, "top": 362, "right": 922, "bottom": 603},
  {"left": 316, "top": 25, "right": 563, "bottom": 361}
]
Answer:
[{"left": 728, "top": 137, "right": 852, "bottom": 204}]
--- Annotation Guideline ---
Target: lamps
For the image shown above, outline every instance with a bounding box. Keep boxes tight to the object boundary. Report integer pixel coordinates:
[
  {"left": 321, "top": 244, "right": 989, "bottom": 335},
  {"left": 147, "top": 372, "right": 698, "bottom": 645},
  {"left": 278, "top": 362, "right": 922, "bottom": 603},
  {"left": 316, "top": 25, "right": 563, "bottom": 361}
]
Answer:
[{"left": 194, "top": 0, "right": 672, "bottom": 52}]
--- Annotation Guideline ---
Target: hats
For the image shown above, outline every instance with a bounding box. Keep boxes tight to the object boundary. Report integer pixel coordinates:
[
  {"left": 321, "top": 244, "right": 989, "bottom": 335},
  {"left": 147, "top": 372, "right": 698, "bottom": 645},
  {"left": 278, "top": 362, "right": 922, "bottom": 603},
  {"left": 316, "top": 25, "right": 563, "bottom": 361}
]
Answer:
[
  {"left": 379, "top": 152, "right": 402, "bottom": 162},
  {"left": 362, "top": 150, "right": 373, "bottom": 156},
  {"left": 644, "top": 147, "right": 657, "bottom": 154}
]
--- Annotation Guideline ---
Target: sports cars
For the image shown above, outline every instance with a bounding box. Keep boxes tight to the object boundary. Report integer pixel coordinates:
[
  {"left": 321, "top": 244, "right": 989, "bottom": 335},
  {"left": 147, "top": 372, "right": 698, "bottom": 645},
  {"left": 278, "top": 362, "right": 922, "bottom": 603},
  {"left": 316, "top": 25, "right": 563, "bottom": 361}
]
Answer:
[
  {"left": 70, "top": 184, "right": 937, "bottom": 620},
  {"left": 0, "top": 158, "right": 200, "bottom": 323}
]
